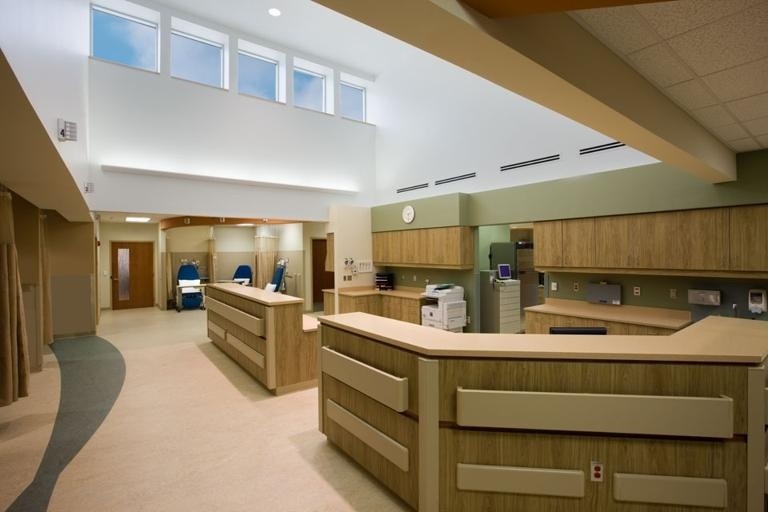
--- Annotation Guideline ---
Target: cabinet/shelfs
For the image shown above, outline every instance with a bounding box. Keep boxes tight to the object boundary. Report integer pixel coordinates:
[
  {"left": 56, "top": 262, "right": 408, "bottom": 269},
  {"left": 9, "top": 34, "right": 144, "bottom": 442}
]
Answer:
[
  {"left": 371, "top": 226, "right": 475, "bottom": 271},
  {"left": 479, "top": 269, "right": 521, "bottom": 333},
  {"left": 355, "top": 294, "right": 380, "bottom": 316},
  {"left": 524, "top": 309, "right": 677, "bottom": 335},
  {"left": 380, "top": 295, "right": 421, "bottom": 325},
  {"left": 533, "top": 204, "right": 768, "bottom": 279}
]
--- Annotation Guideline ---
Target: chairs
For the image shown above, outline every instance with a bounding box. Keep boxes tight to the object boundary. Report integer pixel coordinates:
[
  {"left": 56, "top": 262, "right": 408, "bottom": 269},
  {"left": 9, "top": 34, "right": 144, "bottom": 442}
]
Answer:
[{"left": 175, "top": 263, "right": 285, "bottom": 313}]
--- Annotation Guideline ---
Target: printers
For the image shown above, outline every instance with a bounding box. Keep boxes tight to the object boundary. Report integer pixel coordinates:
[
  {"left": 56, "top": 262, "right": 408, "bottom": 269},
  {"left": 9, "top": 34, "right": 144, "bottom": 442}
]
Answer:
[{"left": 421, "top": 283, "right": 467, "bottom": 330}]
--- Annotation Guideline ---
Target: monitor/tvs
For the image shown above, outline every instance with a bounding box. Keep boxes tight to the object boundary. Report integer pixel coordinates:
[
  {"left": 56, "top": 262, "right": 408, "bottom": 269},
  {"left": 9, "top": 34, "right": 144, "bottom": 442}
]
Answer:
[{"left": 498, "top": 264, "right": 512, "bottom": 279}]
